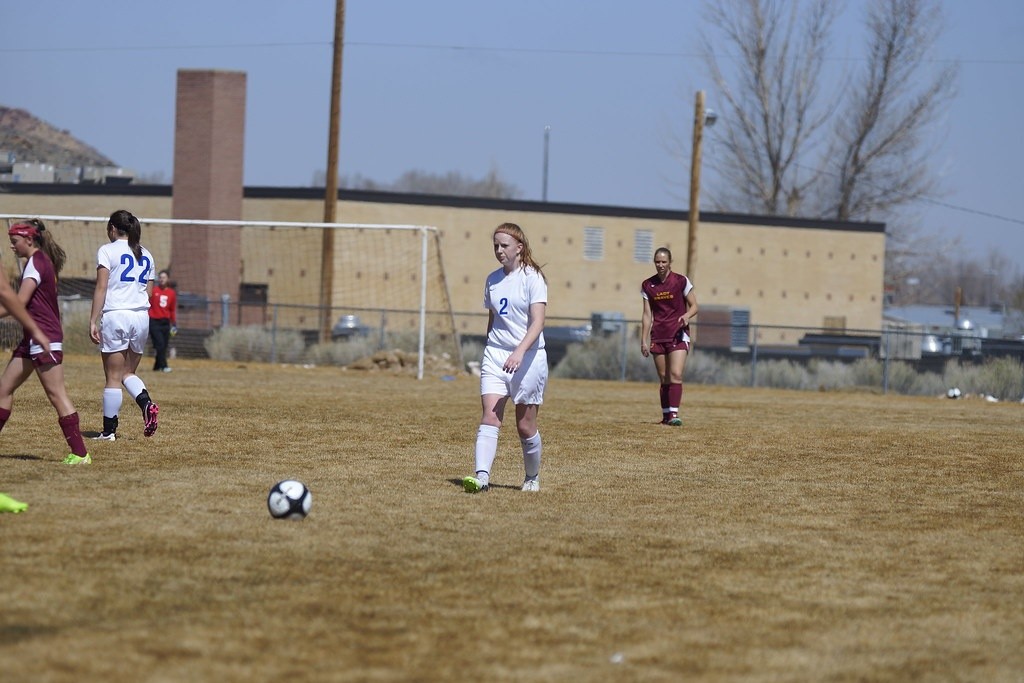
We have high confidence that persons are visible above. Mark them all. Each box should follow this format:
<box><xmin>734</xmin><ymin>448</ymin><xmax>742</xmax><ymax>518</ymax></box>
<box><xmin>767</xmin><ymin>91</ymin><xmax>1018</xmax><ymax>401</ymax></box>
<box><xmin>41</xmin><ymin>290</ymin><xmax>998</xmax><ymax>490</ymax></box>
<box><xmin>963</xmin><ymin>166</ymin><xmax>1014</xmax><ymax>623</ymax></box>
<box><xmin>148</xmin><ymin>270</ymin><xmax>177</xmax><ymax>373</ymax></box>
<box><xmin>463</xmin><ymin>223</ymin><xmax>548</xmax><ymax>494</ymax></box>
<box><xmin>0</xmin><ymin>218</ymin><xmax>91</xmax><ymax>464</ymax></box>
<box><xmin>90</xmin><ymin>210</ymin><xmax>159</xmax><ymax>442</ymax></box>
<box><xmin>0</xmin><ymin>266</ymin><xmax>50</xmax><ymax>356</ymax></box>
<box><xmin>640</xmin><ymin>247</ymin><xmax>698</xmax><ymax>426</ymax></box>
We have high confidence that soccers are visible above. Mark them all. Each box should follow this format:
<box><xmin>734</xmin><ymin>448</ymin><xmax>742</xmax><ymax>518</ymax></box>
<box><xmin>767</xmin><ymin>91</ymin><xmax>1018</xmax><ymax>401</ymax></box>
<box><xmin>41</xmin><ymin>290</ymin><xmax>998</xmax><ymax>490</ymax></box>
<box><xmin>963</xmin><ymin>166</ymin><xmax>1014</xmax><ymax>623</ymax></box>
<box><xmin>267</xmin><ymin>479</ymin><xmax>313</xmax><ymax>522</ymax></box>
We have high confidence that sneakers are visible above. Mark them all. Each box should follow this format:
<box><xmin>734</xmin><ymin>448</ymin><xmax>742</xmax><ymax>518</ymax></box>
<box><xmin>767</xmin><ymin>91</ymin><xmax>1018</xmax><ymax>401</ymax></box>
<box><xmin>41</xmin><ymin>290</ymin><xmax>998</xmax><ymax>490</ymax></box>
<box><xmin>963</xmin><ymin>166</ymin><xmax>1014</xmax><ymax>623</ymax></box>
<box><xmin>91</xmin><ymin>432</ymin><xmax>116</xmax><ymax>441</ymax></box>
<box><xmin>660</xmin><ymin>418</ymin><xmax>682</xmax><ymax>426</ymax></box>
<box><xmin>142</xmin><ymin>401</ymin><xmax>159</xmax><ymax>437</ymax></box>
<box><xmin>522</xmin><ymin>480</ymin><xmax>540</xmax><ymax>492</ymax></box>
<box><xmin>61</xmin><ymin>452</ymin><xmax>92</xmax><ymax>465</ymax></box>
<box><xmin>158</xmin><ymin>366</ymin><xmax>173</xmax><ymax>373</ymax></box>
<box><xmin>0</xmin><ymin>492</ymin><xmax>29</xmax><ymax>514</ymax></box>
<box><xmin>463</xmin><ymin>476</ymin><xmax>489</xmax><ymax>494</ymax></box>
